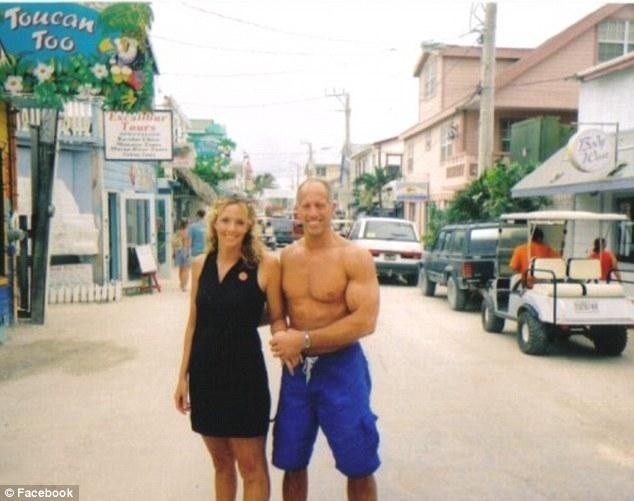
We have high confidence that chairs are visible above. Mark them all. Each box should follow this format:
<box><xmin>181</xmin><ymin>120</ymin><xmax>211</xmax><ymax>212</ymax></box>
<box><xmin>530</xmin><ymin>257</ymin><xmax>624</xmax><ymax>298</ymax></box>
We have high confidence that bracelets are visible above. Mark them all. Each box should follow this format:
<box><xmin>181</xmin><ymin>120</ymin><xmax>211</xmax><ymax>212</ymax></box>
<box><xmin>304</xmin><ymin>330</ymin><xmax>311</xmax><ymax>354</ymax></box>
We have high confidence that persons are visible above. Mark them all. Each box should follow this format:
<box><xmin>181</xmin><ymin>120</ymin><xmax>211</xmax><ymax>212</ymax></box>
<box><xmin>255</xmin><ymin>220</ymin><xmax>274</xmax><ymax>236</ymax></box>
<box><xmin>589</xmin><ymin>238</ymin><xmax>620</xmax><ymax>280</ymax></box>
<box><xmin>175</xmin><ymin>195</ymin><xmax>305</xmax><ymax>501</ymax></box>
<box><xmin>174</xmin><ymin>210</ymin><xmax>210</xmax><ymax>291</ymax></box>
<box><xmin>257</xmin><ymin>177</ymin><xmax>381</xmax><ymax>501</ymax></box>
<box><xmin>509</xmin><ymin>227</ymin><xmax>561</xmax><ymax>289</ymax></box>
